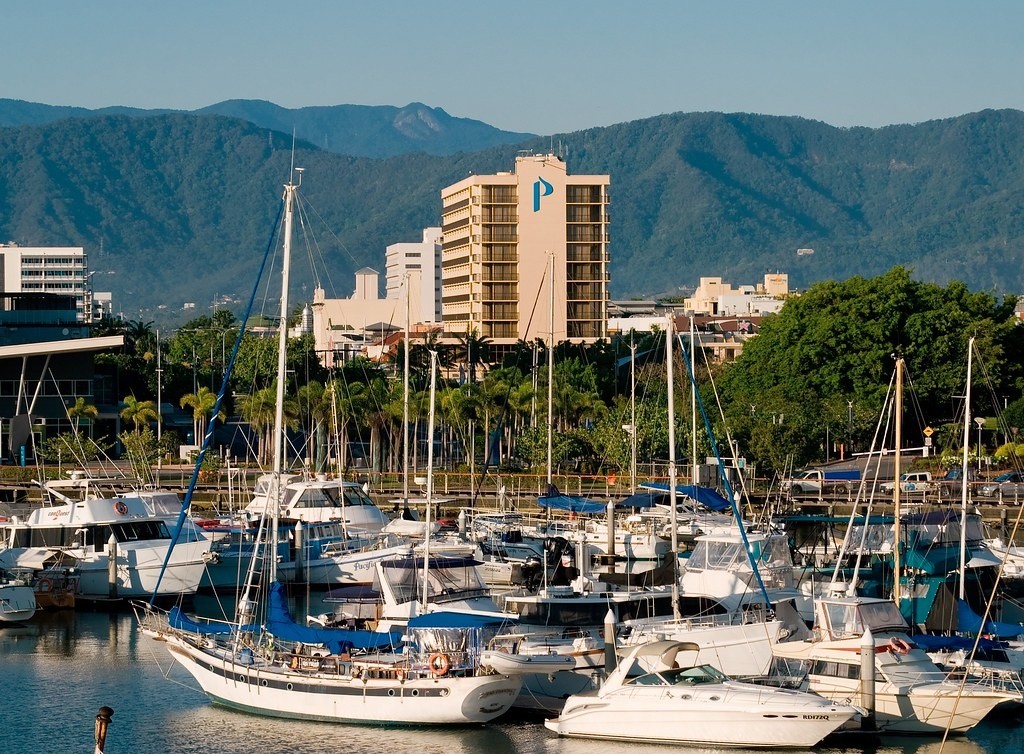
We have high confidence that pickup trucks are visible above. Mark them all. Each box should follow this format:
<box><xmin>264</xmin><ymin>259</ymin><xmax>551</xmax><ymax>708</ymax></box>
<box><xmin>925</xmin><ymin>467</ymin><xmax>987</xmax><ymax>496</ymax></box>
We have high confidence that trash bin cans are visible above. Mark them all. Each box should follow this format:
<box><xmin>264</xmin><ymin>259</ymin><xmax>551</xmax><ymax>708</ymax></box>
<box><xmin>188</xmin><ymin>449</ymin><xmax>200</xmax><ymax>465</ymax></box>
<box><xmin>607</xmin><ymin>469</ymin><xmax>616</xmax><ymax>487</ymax></box>
<box><xmin>240</xmin><ymin>648</ymin><xmax>254</xmax><ymax>664</ymax></box>
<box><xmin>689</xmin><ymin>464</ymin><xmax>710</xmax><ymax>488</ymax></box>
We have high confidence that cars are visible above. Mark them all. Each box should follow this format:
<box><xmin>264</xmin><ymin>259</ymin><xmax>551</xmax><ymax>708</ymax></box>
<box><xmin>982</xmin><ymin>470</ymin><xmax>1024</xmax><ymax>499</ymax></box>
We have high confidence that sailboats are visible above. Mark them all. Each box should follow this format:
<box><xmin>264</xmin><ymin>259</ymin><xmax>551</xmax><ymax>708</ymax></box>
<box><xmin>0</xmin><ymin>165</ymin><xmax>1024</xmax><ymax>738</ymax></box>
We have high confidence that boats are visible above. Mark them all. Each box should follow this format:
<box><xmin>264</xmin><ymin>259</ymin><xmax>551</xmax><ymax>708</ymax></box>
<box><xmin>543</xmin><ymin>639</ymin><xmax>871</xmax><ymax>750</ymax></box>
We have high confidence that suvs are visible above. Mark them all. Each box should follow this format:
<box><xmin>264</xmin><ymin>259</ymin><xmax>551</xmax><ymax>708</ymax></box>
<box><xmin>878</xmin><ymin>471</ymin><xmax>934</xmax><ymax>496</ymax></box>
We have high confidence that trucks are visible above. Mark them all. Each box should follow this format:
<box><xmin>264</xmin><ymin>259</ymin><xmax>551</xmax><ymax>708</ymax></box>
<box><xmin>778</xmin><ymin>470</ymin><xmax>862</xmax><ymax>496</ymax></box>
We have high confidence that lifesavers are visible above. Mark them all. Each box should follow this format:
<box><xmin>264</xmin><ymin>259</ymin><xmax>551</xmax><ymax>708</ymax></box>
<box><xmin>114</xmin><ymin>501</ymin><xmax>128</xmax><ymax>515</ymax></box>
<box><xmin>39</xmin><ymin>578</ymin><xmax>52</xmax><ymax>592</ymax></box>
<box><xmin>889</xmin><ymin>636</ymin><xmax>911</xmax><ymax>654</ymax></box>
<box><xmin>429</xmin><ymin>653</ymin><xmax>449</xmax><ymax>675</ymax></box>
